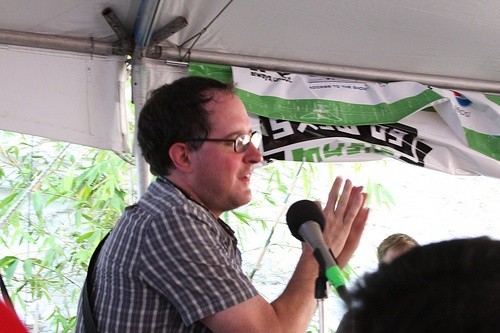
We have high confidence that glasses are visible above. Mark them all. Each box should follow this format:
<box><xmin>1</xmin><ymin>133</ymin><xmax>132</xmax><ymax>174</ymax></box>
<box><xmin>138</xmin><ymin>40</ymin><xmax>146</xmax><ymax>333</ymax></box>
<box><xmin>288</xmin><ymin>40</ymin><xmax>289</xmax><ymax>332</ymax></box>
<box><xmin>178</xmin><ymin>131</ymin><xmax>263</xmax><ymax>153</ymax></box>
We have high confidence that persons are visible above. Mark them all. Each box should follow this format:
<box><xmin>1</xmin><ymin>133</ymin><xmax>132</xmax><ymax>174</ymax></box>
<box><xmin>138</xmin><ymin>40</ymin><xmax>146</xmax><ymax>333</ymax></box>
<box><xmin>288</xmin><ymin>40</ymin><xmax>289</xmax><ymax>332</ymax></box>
<box><xmin>75</xmin><ymin>76</ymin><xmax>370</xmax><ymax>333</ymax></box>
<box><xmin>337</xmin><ymin>234</ymin><xmax>500</xmax><ymax>333</ymax></box>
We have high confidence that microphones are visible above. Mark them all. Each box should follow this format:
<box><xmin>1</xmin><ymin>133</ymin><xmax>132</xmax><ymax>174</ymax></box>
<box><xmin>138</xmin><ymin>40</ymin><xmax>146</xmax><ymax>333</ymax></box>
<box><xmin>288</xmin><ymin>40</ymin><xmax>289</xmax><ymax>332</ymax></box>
<box><xmin>286</xmin><ymin>200</ymin><xmax>354</xmax><ymax>311</ymax></box>
